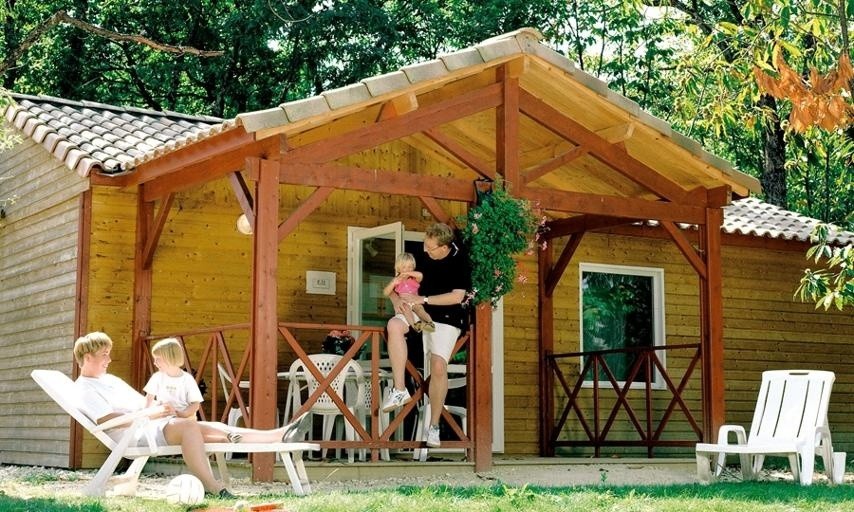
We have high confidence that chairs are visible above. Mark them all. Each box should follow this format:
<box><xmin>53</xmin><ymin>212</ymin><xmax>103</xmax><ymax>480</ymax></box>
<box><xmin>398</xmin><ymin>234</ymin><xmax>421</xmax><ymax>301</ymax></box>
<box><xmin>216</xmin><ymin>353</ymin><xmax>469</xmax><ymax>466</ymax></box>
<box><xmin>695</xmin><ymin>370</ymin><xmax>838</xmax><ymax>487</ymax></box>
<box><xmin>30</xmin><ymin>369</ymin><xmax>323</xmax><ymax>499</ymax></box>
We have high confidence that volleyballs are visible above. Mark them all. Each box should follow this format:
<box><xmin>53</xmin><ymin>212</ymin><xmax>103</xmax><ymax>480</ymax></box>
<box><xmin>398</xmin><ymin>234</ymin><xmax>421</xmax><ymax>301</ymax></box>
<box><xmin>167</xmin><ymin>475</ymin><xmax>204</xmax><ymax>507</ymax></box>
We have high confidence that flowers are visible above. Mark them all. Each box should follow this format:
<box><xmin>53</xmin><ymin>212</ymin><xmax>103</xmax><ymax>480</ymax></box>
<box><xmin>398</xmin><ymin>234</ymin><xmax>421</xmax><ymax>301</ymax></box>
<box><xmin>449</xmin><ymin>175</ymin><xmax>553</xmax><ymax>313</ymax></box>
<box><xmin>320</xmin><ymin>330</ymin><xmax>355</xmax><ymax>355</ymax></box>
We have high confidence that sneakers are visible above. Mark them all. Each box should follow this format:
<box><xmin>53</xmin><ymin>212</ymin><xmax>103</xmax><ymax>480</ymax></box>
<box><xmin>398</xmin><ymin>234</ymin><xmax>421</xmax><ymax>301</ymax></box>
<box><xmin>382</xmin><ymin>386</ymin><xmax>413</xmax><ymax>413</ymax></box>
<box><xmin>413</xmin><ymin>320</ymin><xmax>423</xmax><ymax>334</ymax></box>
<box><xmin>422</xmin><ymin>320</ymin><xmax>435</xmax><ymax>333</ymax></box>
<box><xmin>204</xmin><ymin>488</ymin><xmax>240</xmax><ymax>501</ymax></box>
<box><xmin>425</xmin><ymin>424</ymin><xmax>441</xmax><ymax>448</ymax></box>
<box><xmin>281</xmin><ymin>411</ymin><xmax>312</xmax><ymax>443</ymax></box>
<box><xmin>226</xmin><ymin>433</ymin><xmax>243</xmax><ymax>443</ymax></box>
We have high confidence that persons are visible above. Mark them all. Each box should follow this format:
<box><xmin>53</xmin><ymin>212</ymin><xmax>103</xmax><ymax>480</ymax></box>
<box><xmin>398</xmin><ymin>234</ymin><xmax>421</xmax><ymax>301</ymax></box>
<box><xmin>138</xmin><ymin>336</ymin><xmax>243</xmax><ymax>442</ymax></box>
<box><xmin>381</xmin><ymin>222</ymin><xmax>468</xmax><ymax>449</ymax></box>
<box><xmin>381</xmin><ymin>251</ymin><xmax>436</xmax><ymax>334</ymax></box>
<box><xmin>71</xmin><ymin>329</ymin><xmax>312</xmax><ymax>502</ymax></box>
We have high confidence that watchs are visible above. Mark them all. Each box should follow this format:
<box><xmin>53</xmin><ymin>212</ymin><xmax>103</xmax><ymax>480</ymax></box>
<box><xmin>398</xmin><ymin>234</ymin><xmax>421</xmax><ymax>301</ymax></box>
<box><xmin>422</xmin><ymin>293</ymin><xmax>430</xmax><ymax>305</ymax></box>
